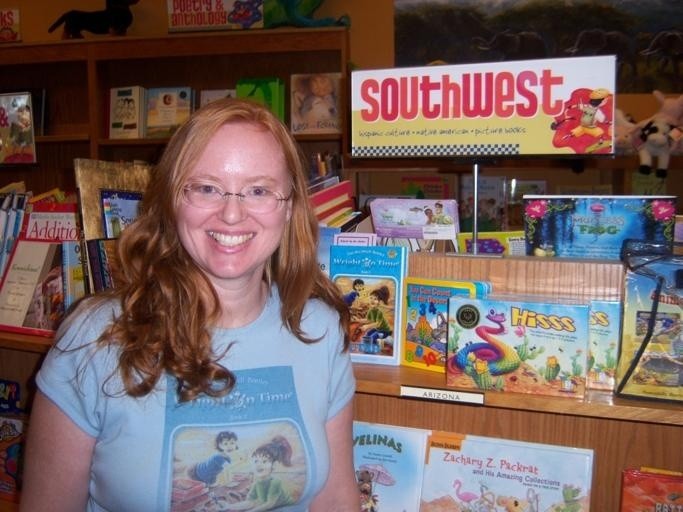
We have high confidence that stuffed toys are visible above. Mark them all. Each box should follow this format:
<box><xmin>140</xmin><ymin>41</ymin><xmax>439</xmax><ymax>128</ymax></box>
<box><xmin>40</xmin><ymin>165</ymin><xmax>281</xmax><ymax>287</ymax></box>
<box><xmin>48</xmin><ymin>0</ymin><xmax>138</xmax><ymax>42</ymax></box>
<box><xmin>634</xmin><ymin>88</ymin><xmax>682</xmax><ymax>177</ymax></box>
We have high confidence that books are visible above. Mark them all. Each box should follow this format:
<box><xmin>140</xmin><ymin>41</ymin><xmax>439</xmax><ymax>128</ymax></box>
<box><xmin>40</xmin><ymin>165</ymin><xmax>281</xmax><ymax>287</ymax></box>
<box><xmin>0</xmin><ymin>5</ymin><xmax>47</xmax><ymax>167</ymax></box>
<box><xmin>106</xmin><ymin>70</ymin><xmax>340</xmax><ymax>142</ymax></box>
<box><xmin>166</xmin><ymin>0</ymin><xmax>264</xmax><ymax>33</ymax></box>
<box><xmin>0</xmin><ymin>177</ymin><xmax>683</xmax><ymax>510</ymax></box>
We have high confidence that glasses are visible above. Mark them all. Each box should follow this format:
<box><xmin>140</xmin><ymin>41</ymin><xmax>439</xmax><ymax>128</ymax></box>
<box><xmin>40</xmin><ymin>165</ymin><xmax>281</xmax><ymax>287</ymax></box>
<box><xmin>178</xmin><ymin>181</ymin><xmax>294</xmax><ymax>215</ymax></box>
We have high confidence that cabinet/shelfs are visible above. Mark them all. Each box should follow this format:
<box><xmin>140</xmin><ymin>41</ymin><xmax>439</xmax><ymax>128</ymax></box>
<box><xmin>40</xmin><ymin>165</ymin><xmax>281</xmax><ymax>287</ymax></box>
<box><xmin>1</xmin><ymin>1</ymin><xmax>394</xmax><ymax>219</ymax></box>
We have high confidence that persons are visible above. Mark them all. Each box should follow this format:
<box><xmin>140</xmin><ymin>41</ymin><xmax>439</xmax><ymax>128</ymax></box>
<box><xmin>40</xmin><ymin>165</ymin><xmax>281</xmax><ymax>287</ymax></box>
<box><xmin>18</xmin><ymin>98</ymin><xmax>360</xmax><ymax>511</ymax></box>
